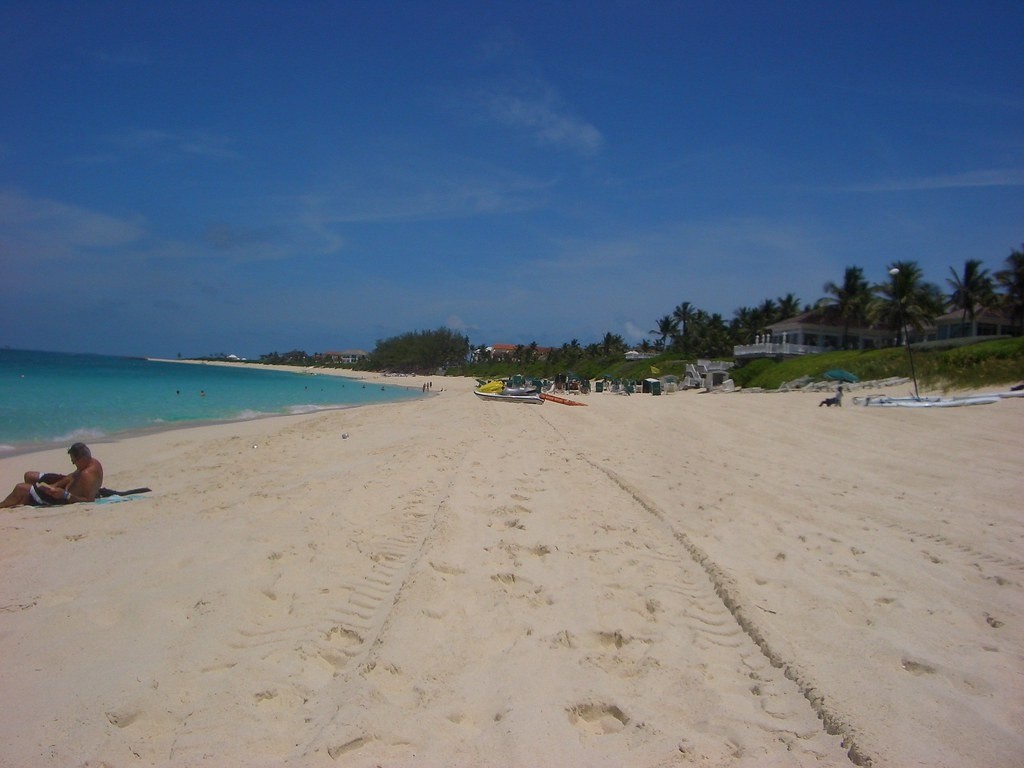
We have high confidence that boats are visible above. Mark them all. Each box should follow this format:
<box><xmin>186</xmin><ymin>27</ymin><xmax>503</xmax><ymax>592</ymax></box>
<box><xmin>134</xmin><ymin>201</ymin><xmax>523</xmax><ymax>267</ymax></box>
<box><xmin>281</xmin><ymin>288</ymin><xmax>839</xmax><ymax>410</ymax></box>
<box><xmin>474</xmin><ymin>391</ymin><xmax>545</xmax><ymax>405</ymax></box>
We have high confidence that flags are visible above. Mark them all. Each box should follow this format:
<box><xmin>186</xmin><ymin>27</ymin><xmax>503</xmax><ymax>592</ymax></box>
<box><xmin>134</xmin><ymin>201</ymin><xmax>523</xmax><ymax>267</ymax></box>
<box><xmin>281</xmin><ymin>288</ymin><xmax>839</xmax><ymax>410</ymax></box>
<box><xmin>651</xmin><ymin>367</ymin><xmax>659</xmax><ymax>374</ymax></box>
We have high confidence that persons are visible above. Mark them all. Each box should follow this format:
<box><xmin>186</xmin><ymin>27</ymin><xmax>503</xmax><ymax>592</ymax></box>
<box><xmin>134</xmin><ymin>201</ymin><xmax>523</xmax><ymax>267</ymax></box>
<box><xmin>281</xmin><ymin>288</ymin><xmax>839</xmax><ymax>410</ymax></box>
<box><xmin>819</xmin><ymin>386</ymin><xmax>843</xmax><ymax>407</ymax></box>
<box><xmin>176</xmin><ymin>390</ymin><xmax>180</xmax><ymax>394</ymax></box>
<box><xmin>304</xmin><ymin>381</ymin><xmax>432</xmax><ymax>393</ymax></box>
<box><xmin>200</xmin><ymin>390</ymin><xmax>205</xmax><ymax>396</ymax></box>
<box><xmin>0</xmin><ymin>442</ymin><xmax>103</xmax><ymax>508</ymax></box>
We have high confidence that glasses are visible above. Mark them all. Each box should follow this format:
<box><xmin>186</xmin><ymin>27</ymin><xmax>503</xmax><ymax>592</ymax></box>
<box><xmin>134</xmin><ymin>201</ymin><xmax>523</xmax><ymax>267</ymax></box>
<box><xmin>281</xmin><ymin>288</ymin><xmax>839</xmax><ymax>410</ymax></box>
<box><xmin>73</xmin><ymin>456</ymin><xmax>80</xmax><ymax>464</ymax></box>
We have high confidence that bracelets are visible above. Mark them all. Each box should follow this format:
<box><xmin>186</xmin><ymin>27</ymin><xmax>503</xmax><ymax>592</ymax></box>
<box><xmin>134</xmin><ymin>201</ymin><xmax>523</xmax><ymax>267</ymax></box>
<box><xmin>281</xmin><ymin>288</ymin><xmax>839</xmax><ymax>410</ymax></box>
<box><xmin>64</xmin><ymin>492</ymin><xmax>72</xmax><ymax>499</ymax></box>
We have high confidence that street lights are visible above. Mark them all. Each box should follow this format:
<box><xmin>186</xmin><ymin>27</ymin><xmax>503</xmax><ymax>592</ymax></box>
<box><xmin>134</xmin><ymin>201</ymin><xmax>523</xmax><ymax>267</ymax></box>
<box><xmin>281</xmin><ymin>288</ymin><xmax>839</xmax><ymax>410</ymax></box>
<box><xmin>889</xmin><ymin>266</ymin><xmax>920</xmax><ymax>403</ymax></box>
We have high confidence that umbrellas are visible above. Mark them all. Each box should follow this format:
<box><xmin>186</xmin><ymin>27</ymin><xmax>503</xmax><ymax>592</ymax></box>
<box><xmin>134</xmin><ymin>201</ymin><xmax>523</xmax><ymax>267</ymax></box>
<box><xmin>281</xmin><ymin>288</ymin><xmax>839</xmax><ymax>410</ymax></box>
<box><xmin>823</xmin><ymin>368</ymin><xmax>858</xmax><ymax>389</ymax></box>
